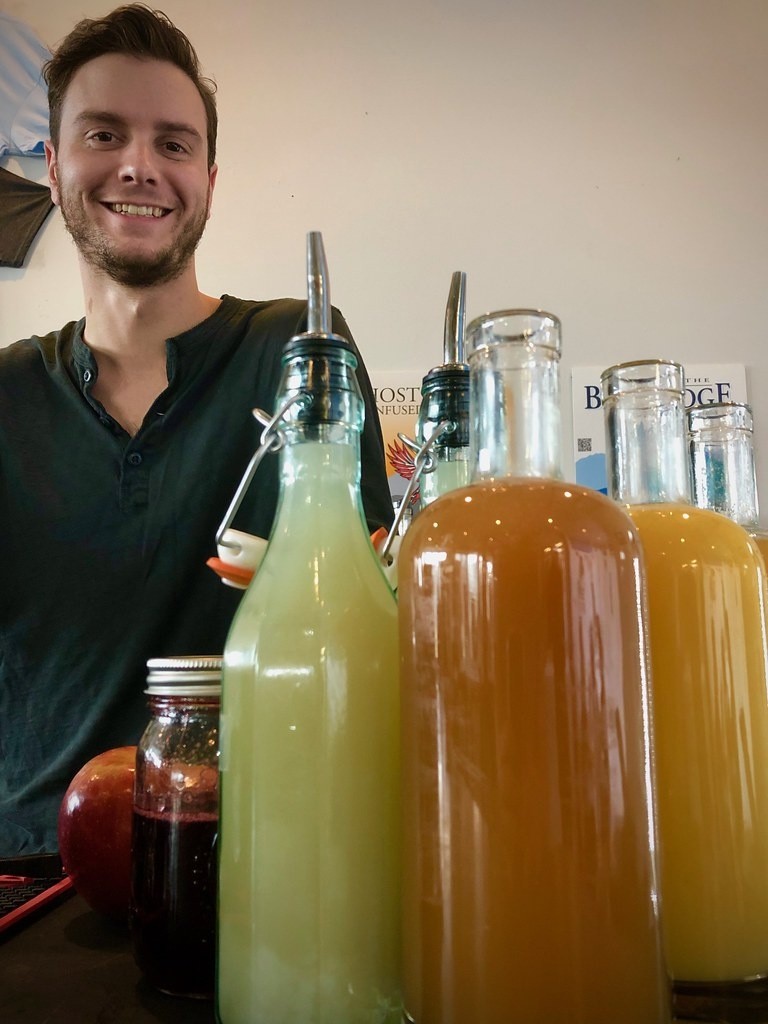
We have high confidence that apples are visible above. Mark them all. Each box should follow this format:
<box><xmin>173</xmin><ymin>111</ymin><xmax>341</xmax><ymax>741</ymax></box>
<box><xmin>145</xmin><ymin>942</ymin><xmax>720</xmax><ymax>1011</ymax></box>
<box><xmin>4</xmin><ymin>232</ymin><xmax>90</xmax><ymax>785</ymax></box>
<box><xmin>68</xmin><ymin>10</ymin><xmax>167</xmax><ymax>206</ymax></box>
<box><xmin>53</xmin><ymin>747</ymin><xmax>219</xmax><ymax>926</ymax></box>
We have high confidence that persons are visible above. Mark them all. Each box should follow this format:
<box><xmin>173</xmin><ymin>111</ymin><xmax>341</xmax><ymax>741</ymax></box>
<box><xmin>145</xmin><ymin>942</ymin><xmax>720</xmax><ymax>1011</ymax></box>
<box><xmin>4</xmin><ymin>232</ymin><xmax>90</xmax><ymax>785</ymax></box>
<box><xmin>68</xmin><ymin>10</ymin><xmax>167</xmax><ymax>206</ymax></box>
<box><xmin>0</xmin><ymin>4</ymin><xmax>398</xmax><ymax>859</ymax></box>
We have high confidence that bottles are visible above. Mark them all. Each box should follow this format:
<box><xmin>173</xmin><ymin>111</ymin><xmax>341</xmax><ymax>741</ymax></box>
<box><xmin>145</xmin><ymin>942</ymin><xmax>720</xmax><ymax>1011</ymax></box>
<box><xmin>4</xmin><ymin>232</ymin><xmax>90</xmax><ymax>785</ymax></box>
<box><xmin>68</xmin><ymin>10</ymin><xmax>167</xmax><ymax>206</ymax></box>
<box><xmin>133</xmin><ymin>654</ymin><xmax>225</xmax><ymax>1003</ymax></box>
<box><xmin>599</xmin><ymin>359</ymin><xmax>768</xmax><ymax>998</ymax></box>
<box><xmin>394</xmin><ymin>311</ymin><xmax>673</xmax><ymax>1024</ymax></box>
<box><xmin>681</xmin><ymin>402</ymin><xmax>768</xmax><ymax>572</ymax></box>
<box><xmin>205</xmin><ymin>230</ymin><xmax>403</xmax><ymax>1024</ymax></box>
<box><xmin>367</xmin><ymin>269</ymin><xmax>501</xmax><ymax>604</ymax></box>
